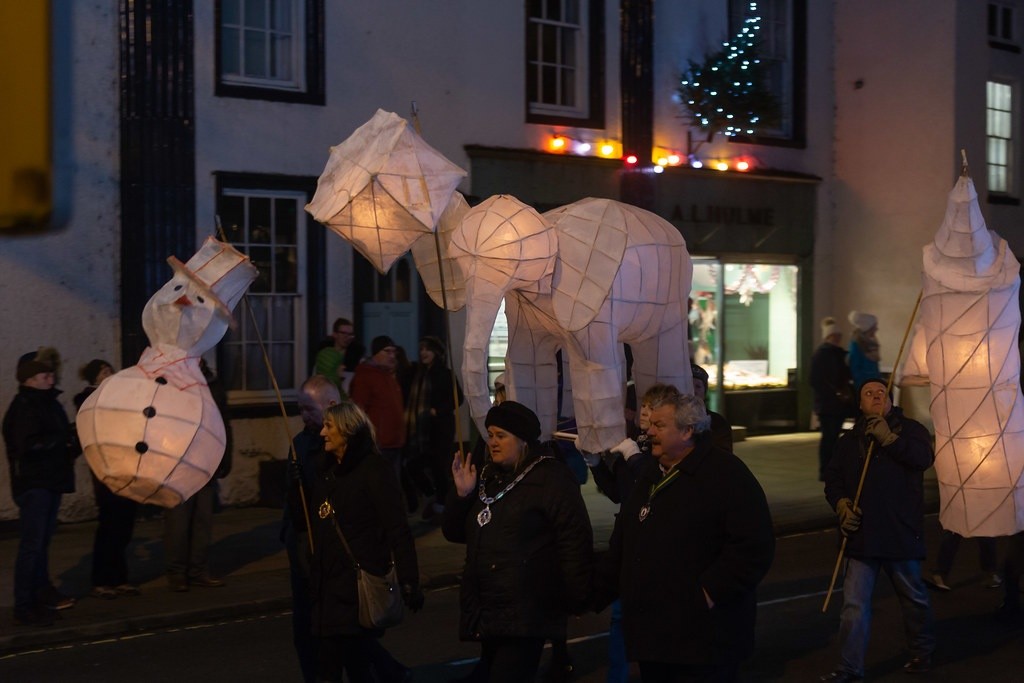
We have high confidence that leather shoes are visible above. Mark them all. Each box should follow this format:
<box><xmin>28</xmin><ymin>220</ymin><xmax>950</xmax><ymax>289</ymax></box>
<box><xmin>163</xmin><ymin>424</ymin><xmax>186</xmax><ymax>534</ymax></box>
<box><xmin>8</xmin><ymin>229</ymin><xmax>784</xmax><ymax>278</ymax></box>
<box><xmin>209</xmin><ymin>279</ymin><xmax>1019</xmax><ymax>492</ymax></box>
<box><xmin>190</xmin><ymin>574</ymin><xmax>228</xmax><ymax>587</ymax></box>
<box><xmin>168</xmin><ymin>578</ymin><xmax>190</xmax><ymax>591</ymax></box>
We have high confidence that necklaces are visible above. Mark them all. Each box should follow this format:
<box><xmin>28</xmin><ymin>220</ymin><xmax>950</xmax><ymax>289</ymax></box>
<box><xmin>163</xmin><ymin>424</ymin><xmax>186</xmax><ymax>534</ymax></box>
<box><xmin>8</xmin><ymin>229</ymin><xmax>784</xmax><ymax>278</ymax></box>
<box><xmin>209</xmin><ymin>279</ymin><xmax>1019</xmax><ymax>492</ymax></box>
<box><xmin>319</xmin><ymin>494</ymin><xmax>330</xmax><ymax>518</ymax></box>
<box><xmin>640</xmin><ymin>470</ymin><xmax>682</xmax><ymax>522</ymax></box>
<box><xmin>477</xmin><ymin>455</ymin><xmax>556</xmax><ymax>528</ymax></box>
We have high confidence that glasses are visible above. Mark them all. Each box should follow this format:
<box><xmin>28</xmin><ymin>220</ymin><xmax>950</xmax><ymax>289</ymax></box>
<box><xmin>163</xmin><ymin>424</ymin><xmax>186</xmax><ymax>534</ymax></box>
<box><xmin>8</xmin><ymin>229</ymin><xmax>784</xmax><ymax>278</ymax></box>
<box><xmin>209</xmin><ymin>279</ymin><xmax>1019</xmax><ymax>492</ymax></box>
<box><xmin>383</xmin><ymin>349</ymin><xmax>399</xmax><ymax>355</ymax></box>
<box><xmin>334</xmin><ymin>330</ymin><xmax>356</xmax><ymax>340</ymax></box>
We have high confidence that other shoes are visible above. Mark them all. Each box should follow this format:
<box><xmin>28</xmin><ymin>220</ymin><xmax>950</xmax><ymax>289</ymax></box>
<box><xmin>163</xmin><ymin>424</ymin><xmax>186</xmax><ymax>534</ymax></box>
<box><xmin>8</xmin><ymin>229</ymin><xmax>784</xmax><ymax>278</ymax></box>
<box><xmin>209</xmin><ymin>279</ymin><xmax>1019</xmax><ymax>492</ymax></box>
<box><xmin>13</xmin><ymin>609</ymin><xmax>53</xmax><ymax>627</ymax></box>
<box><xmin>40</xmin><ymin>589</ymin><xmax>74</xmax><ymax>609</ymax></box>
<box><xmin>113</xmin><ymin>584</ymin><xmax>138</xmax><ymax>597</ymax></box>
<box><xmin>902</xmin><ymin>655</ymin><xmax>932</xmax><ymax>672</ymax></box>
<box><xmin>928</xmin><ymin>575</ymin><xmax>951</xmax><ymax>592</ymax></box>
<box><xmin>91</xmin><ymin>587</ymin><xmax>120</xmax><ymax>599</ymax></box>
<box><xmin>818</xmin><ymin>670</ymin><xmax>865</xmax><ymax>683</ymax></box>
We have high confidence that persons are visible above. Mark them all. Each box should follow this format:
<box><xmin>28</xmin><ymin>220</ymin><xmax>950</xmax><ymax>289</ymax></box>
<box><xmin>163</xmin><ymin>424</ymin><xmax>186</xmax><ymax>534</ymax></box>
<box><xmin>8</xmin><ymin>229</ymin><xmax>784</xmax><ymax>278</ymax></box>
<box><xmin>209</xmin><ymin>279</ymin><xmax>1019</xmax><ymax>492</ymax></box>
<box><xmin>314</xmin><ymin>317</ymin><xmax>465</xmax><ymax>516</ymax></box>
<box><xmin>807</xmin><ymin>309</ymin><xmax>882</xmax><ymax>486</ymax></box>
<box><xmin>163</xmin><ymin>356</ymin><xmax>233</xmax><ymax>592</ymax></box>
<box><xmin>72</xmin><ymin>360</ymin><xmax>140</xmax><ymax>598</ymax></box>
<box><xmin>2</xmin><ymin>348</ymin><xmax>83</xmax><ymax>627</ymax></box>
<box><xmin>278</xmin><ymin>375</ymin><xmax>423</xmax><ymax>683</ymax></box>
<box><xmin>441</xmin><ymin>382</ymin><xmax>776</xmax><ymax>683</ymax></box>
<box><xmin>927</xmin><ymin>530</ymin><xmax>1024</xmax><ymax>616</ymax></box>
<box><xmin>820</xmin><ymin>375</ymin><xmax>935</xmax><ymax>683</ymax></box>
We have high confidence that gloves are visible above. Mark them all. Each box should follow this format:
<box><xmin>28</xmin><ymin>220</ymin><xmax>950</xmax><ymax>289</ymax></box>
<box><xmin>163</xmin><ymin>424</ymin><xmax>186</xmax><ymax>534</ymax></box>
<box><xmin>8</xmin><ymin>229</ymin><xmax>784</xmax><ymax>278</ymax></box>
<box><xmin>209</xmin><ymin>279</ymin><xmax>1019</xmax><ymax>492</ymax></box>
<box><xmin>283</xmin><ymin>461</ymin><xmax>304</xmax><ymax>484</ymax></box>
<box><xmin>835</xmin><ymin>497</ymin><xmax>863</xmax><ymax>538</ymax></box>
<box><xmin>405</xmin><ymin>586</ymin><xmax>425</xmax><ymax>614</ymax></box>
<box><xmin>864</xmin><ymin>414</ymin><xmax>899</xmax><ymax>445</ymax></box>
<box><xmin>610</xmin><ymin>438</ymin><xmax>642</xmax><ymax>462</ymax></box>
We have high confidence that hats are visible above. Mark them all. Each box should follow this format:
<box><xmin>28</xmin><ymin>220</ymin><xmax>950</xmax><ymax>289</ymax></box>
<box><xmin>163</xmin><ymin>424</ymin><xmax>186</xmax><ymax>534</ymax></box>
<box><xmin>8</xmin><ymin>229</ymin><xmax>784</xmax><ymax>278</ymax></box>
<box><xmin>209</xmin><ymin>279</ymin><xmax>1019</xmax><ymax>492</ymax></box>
<box><xmin>371</xmin><ymin>336</ymin><xmax>397</xmax><ymax>356</ymax></box>
<box><xmin>421</xmin><ymin>335</ymin><xmax>444</xmax><ymax>356</ymax></box>
<box><xmin>484</xmin><ymin>401</ymin><xmax>541</xmax><ymax>448</ymax></box>
<box><xmin>17</xmin><ymin>347</ymin><xmax>57</xmax><ymax>384</ymax></box>
<box><xmin>79</xmin><ymin>359</ymin><xmax>110</xmax><ymax>385</ymax></box>
<box><xmin>821</xmin><ymin>317</ymin><xmax>844</xmax><ymax>340</ymax></box>
<box><xmin>848</xmin><ymin>311</ymin><xmax>876</xmax><ymax>334</ymax></box>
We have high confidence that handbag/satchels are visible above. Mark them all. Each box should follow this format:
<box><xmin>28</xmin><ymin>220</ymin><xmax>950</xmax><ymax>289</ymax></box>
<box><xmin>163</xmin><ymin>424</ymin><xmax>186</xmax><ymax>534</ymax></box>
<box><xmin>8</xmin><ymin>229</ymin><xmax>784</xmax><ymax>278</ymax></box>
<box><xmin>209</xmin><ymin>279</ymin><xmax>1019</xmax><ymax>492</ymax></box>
<box><xmin>355</xmin><ymin>566</ymin><xmax>405</xmax><ymax>628</ymax></box>
